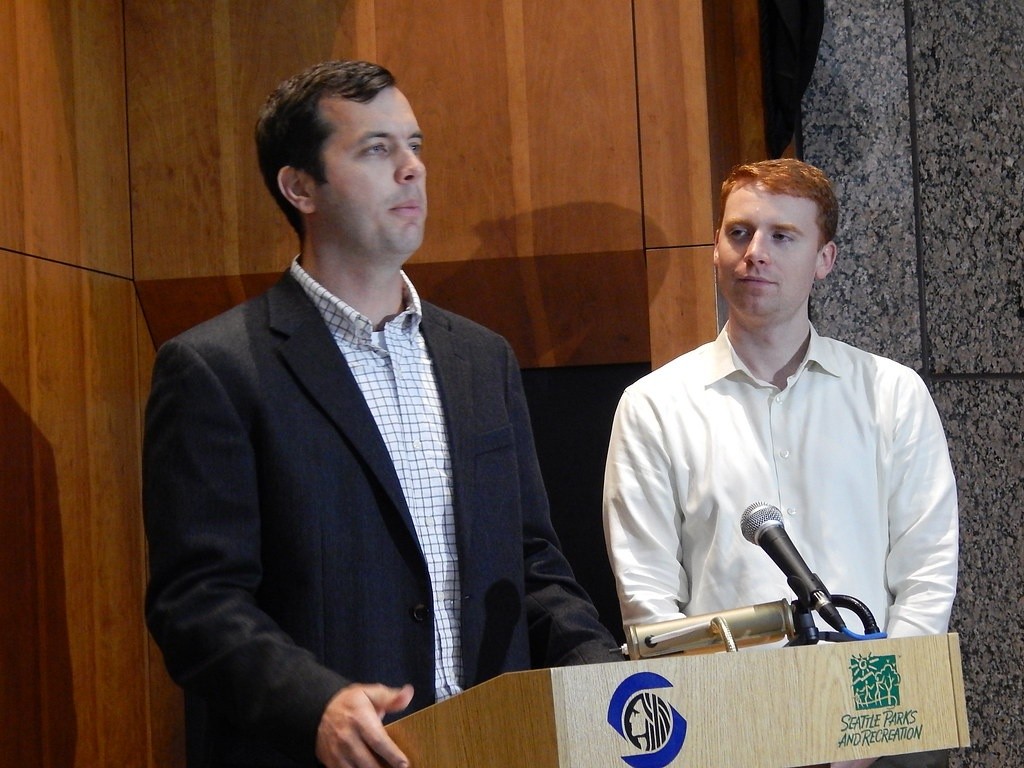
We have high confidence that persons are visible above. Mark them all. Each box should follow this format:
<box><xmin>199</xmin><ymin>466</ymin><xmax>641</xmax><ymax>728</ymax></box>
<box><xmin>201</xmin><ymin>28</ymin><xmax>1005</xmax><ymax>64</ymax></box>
<box><xmin>604</xmin><ymin>156</ymin><xmax>960</xmax><ymax>768</ymax></box>
<box><xmin>141</xmin><ymin>60</ymin><xmax>623</xmax><ymax>768</ymax></box>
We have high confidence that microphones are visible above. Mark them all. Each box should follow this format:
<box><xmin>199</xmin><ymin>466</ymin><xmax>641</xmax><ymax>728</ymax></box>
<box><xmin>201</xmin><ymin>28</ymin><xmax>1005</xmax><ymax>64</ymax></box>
<box><xmin>740</xmin><ymin>502</ymin><xmax>845</xmax><ymax>633</ymax></box>
